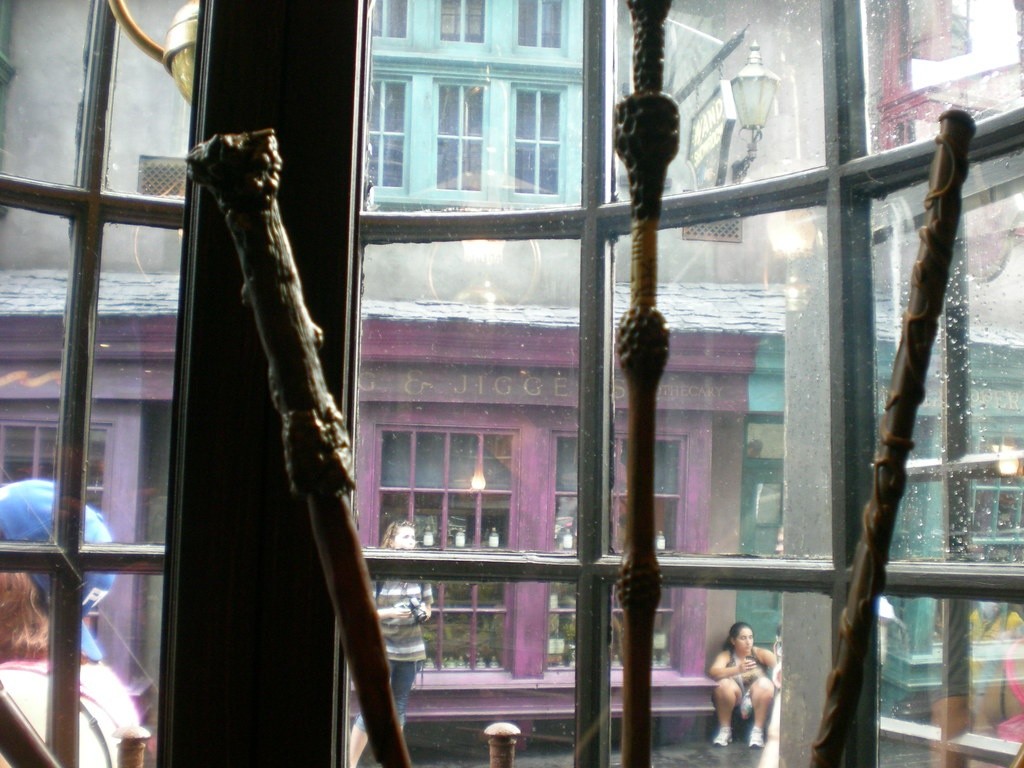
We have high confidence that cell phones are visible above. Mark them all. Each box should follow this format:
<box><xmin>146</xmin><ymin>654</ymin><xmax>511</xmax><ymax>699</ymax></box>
<box><xmin>746</xmin><ymin>656</ymin><xmax>754</xmax><ymax>662</ymax></box>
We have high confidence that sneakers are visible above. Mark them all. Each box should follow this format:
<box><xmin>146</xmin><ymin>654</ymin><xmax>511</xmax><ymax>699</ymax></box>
<box><xmin>713</xmin><ymin>727</ymin><xmax>732</xmax><ymax>745</ymax></box>
<box><xmin>749</xmin><ymin>727</ymin><xmax>765</xmax><ymax>747</ymax></box>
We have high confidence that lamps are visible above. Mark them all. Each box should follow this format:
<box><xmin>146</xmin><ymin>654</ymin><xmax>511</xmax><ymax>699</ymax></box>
<box><xmin>563</xmin><ymin>527</ymin><xmax>572</xmax><ymax>549</ymax></box>
<box><xmin>728</xmin><ymin>36</ymin><xmax>780</xmax><ymax>185</ymax></box>
<box><xmin>488</xmin><ymin>526</ymin><xmax>499</xmax><ymax>546</ymax></box>
<box><xmin>422</xmin><ymin>524</ymin><xmax>436</xmax><ymax>545</ymax></box>
<box><xmin>102</xmin><ymin>0</ymin><xmax>200</xmax><ymax>106</ymax></box>
<box><xmin>454</xmin><ymin>526</ymin><xmax>466</xmax><ymax>546</ymax></box>
<box><xmin>656</xmin><ymin>531</ymin><xmax>666</xmax><ymax>550</ymax></box>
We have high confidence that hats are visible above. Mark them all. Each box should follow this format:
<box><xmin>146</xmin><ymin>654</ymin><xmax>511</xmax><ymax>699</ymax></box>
<box><xmin>0</xmin><ymin>480</ymin><xmax>114</xmax><ymax>660</ymax></box>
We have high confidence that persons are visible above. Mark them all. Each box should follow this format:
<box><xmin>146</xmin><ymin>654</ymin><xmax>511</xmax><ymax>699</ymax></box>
<box><xmin>708</xmin><ymin>622</ymin><xmax>779</xmax><ymax>751</ymax></box>
<box><xmin>926</xmin><ymin>598</ymin><xmax>1024</xmax><ymax>767</ymax></box>
<box><xmin>347</xmin><ymin>518</ymin><xmax>436</xmax><ymax>767</ymax></box>
<box><xmin>0</xmin><ymin>478</ymin><xmax>148</xmax><ymax>768</ymax></box>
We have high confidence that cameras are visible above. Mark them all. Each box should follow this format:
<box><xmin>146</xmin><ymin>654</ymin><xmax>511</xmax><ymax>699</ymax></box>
<box><xmin>399</xmin><ymin>596</ymin><xmax>427</xmax><ymax>623</ymax></box>
